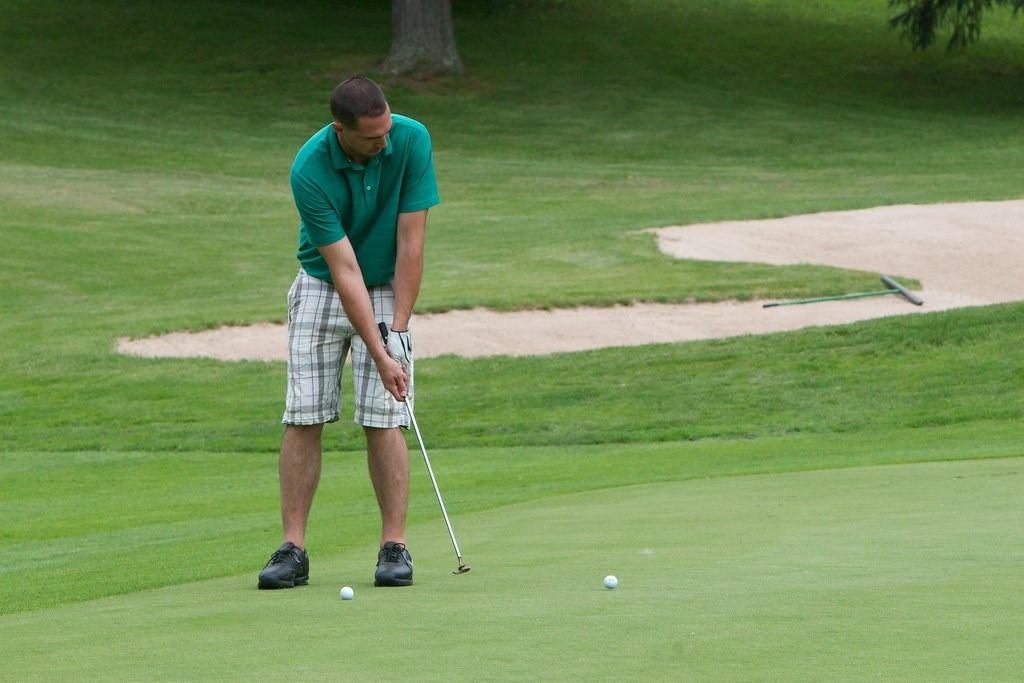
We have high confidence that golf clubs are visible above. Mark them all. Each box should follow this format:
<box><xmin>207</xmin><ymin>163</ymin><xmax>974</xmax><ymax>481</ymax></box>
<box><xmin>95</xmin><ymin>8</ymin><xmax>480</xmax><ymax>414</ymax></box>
<box><xmin>378</xmin><ymin>318</ymin><xmax>471</xmax><ymax>575</ymax></box>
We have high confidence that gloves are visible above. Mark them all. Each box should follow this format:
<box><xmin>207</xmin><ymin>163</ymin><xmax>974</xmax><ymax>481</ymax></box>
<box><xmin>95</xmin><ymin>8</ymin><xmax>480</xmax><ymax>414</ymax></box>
<box><xmin>387</xmin><ymin>327</ymin><xmax>412</xmax><ymax>375</ymax></box>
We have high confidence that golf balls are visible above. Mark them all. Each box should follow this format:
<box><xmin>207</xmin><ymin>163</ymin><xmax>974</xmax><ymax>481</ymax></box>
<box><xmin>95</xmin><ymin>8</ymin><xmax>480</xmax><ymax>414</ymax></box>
<box><xmin>340</xmin><ymin>587</ymin><xmax>354</xmax><ymax>600</ymax></box>
<box><xmin>604</xmin><ymin>574</ymin><xmax>618</xmax><ymax>589</ymax></box>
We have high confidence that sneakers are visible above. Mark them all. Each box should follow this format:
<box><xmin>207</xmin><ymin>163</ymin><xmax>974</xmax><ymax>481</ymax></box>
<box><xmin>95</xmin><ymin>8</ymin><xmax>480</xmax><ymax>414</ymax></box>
<box><xmin>374</xmin><ymin>541</ymin><xmax>413</xmax><ymax>587</ymax></box>
<box><xmin>257</xmin><ymin>542</ymin><xmax>309</xmax><ymax>590</ymax></box>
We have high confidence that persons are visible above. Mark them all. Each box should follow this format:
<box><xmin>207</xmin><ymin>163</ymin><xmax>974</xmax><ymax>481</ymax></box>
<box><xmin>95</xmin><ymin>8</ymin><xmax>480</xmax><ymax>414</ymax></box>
<box><xmin>257</xmin><ymin>78</ymin><xmax>440</xmax><ymax>585</ymax></box>
